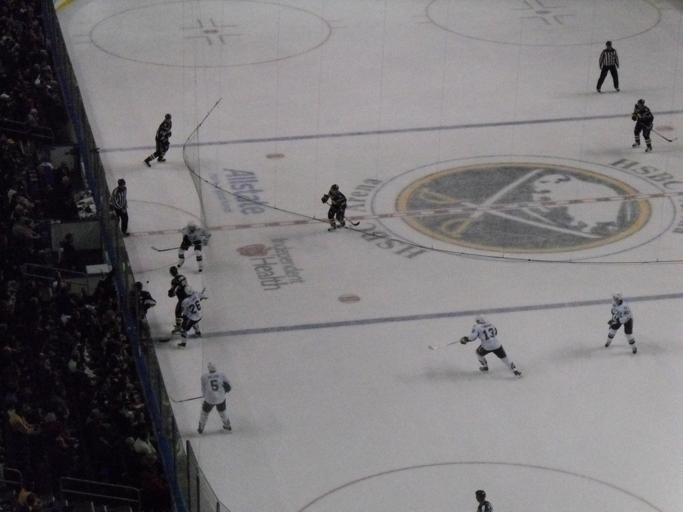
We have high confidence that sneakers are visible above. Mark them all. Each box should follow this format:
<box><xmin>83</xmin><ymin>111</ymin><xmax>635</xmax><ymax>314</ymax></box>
<box><xmin>196</xmin><ymin>428</ymin><xmax>204</xmax><ymax>433</ymax></box>
<box><xmin>122</xmin><ymin>231</ymin><xmax>130</xmax><ymax>236</ymax></box>
<box><xmin>514</xmin><ymin>371</ymin><xmax>522</xmax><ymax>376</ymax></box>
<box><xmin>194</xmin><ymin>331</ymin><xmax>201</xmax><ymax>335</ymax></box>
<box><xmin>329</xmin><ymin>221</ymin><xmax>345</xmax><ymax>230</ymax></box>
<box><xmin>199</xmin><ymin>269</ymin><xmax>202</xmax><ymax>272</ymax></box>
<box><xmin>223</xmin><ymin>425</ymin><xmax>231</xmax><ymax>431</ymax></box>
<box><xmin>632</xmin><ymin>345</ymin><xmax>638</xmax><ymax>354</ymax></box>
<box><xmin>632</xmin><ymin>142</ymin><xmax>652</xmax><ymax>152</ymax></box>
<box><xmin>178</xmin><ymin>343</ymin><xmax>186</xmax><ymax>347</ymax></box>
<box><xmin>614</xmin><ymin>87</ymin><xmax>619</xmax><ymax>91</ymax></box>
<box><xmin>605</xmin><ymin>341</ymin><xmax>612</xmax><ymax>347</ymax></box>
<box><xmin>178</xmin><ymin>261</ymin><xmax>184</xmax><ymax>267</ymax></box>
<box><xmin>479</xmin><ymin>366</ymin><xmax>488</xmax><ymax>371</ymax></box>
<box><xmin>597</xmin><ymin>88</ymin><xmax>601</xmax><ymax>92</ymax></box>
<box><xmin>157</xmin><ymin>157</ymin><xmax>166</xmax><ymax>162</ymax></box>
<box><xmin>145</xmin><ymin>159</ymin><xmax>151</xmax><ymax>167</ymax></box>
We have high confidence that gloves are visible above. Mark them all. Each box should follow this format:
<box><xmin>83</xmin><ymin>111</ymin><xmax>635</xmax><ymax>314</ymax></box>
<box><xmin>321</xmin><ymin>193</ymin><xmax>329</xmax><ymax>203</ymax></box>
<box><xmin>460</xmin><ymin>336</ymin><xmax>469</xmax><ymax>344</ymax></box>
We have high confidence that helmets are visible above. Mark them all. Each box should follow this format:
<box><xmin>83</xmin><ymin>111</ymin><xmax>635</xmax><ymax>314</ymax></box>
<box><xmin>188</xmin><ymin>220</ymin><xmax>196</xmax><ymax>229</ymax></box>
<box><xmin>613</xmin><ymin>291</ymin><xmax>623</xmax><ymax>301</ymax></box>
<box><xmin>169</xmin><ymin>266</ymin><xmax>177</xmax><ymax>275</ymax></box>
<box><xmin>118</xmin><ymin>179</ymin><xmax>126</xmax><ymax>186</ymax></box>
<box><xmin>331</xmin><ymin>184</ymin><xmax>340</xmax><ymax>191</ymax></box>
<box><xmin>475</xmin><ymin>490</ymin><xmax>487</xmax><ymax>500</ymax></box>
<box><xmin>605</xmin><ymin>40</ymin><xmax>612</xmax><ymax>46</ymax></box>
<box><xmin>475</xmin><ymin>313</ymin><xmax>486</xmax><ymax>321</ymax></box>
<box><xmin>638</xmin><ymin>99</ymin><xmax>645</xmax><ymax>105</ymax></box>
<box><xmin>165</xmin><ymin>113</ymin><xmax>172</xmax><ymax>120</ymax></box>
<box><xmin>207</xmin><ymin>361</ymin><xmax>217</xmax><ymax>371</ymax></box>
<box><xmin>185</xmin><ymin>286</ymin><xmax>193</xmax><ymax>294</ymax></box>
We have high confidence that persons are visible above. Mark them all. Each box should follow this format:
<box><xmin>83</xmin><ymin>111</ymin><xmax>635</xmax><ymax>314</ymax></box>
<box><xmin>460</xmin><ymin>314</ymin><xmax>522</xmax><ymax>377</ymax></box>
<box><xmin>605</xmin><ymin>293</ymin><xmax>637</xmax><ymax>354</ymax></box>
<box><xmin>177</xmin><ymin>220</ymin><xmax>212</xmax><ymax>273</ymax></box>
<box><xmin>596</xmin><ymin>40</ymin><xmax>620</xmax><ymax>92</ymax></box>
<box><xmin>1</xmin><ymin>278</ymin><xmax>158</xmax><ymax>512</ymax></box>
<box><xmin>321</xmin><ymin>184</ymin><xmax>347</xmax><ymax>231</ymax></box>
<box><xmin>144</xmin><ymin>114</ymin><xmax>172</xmax><ymax>167</ymax></box>
<box><xmin>631</xmin><ymin>99</ymin><xmax>654</xmax><ymax>152</ymax></box>
<box><xmin>197</xmin><ymin>360</ymin><xmax>233</xmax><ymax>434</ymax></box>
<box><xmin>0</xmin><ymin>1</ymin><xmax>83</xmax><ymax>283</ymax></box>
<box><xmin>111</xmin><ymin>179</ymin><xmax>130</xmax><ymax>236</ymax></box>
<box><xmin>168</xmin><ymin>266</ymin><xmax>209</xmax><ymax>348</ymax></box>
<box><xmin>475</xmin><ymin>490</ymin><xmax>493</xmax><ymax>512</ymax></box>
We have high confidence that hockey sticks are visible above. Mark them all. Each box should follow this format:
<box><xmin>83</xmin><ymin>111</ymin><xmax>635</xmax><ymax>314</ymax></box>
<box><xmin>200</xmin><ymin>297</ymin><xmax>208</xmax><ymax>301</ymax></box>
<box><xmin>170</xmin><ymin>394</ymin><xmax>203</xmax><ymax>403</ymax></box>
<box><xmin>151</xmin><ymin>245</ymin><xmax>204</xmax><ymax>251</ymax></box>
<box><xmin>632</xmin><ymin>113</ymin><xmax>678</xmax><ymax>142</ymax></box>
<box><xmin>326</xmin><ymin>201</ymin><xmax>360</xmax><ymax>226</ymax></box>
<box><xmin>428</xmin><ymin>341</ymin><xmax>461</xmax><ymax>351</ymax></box>
